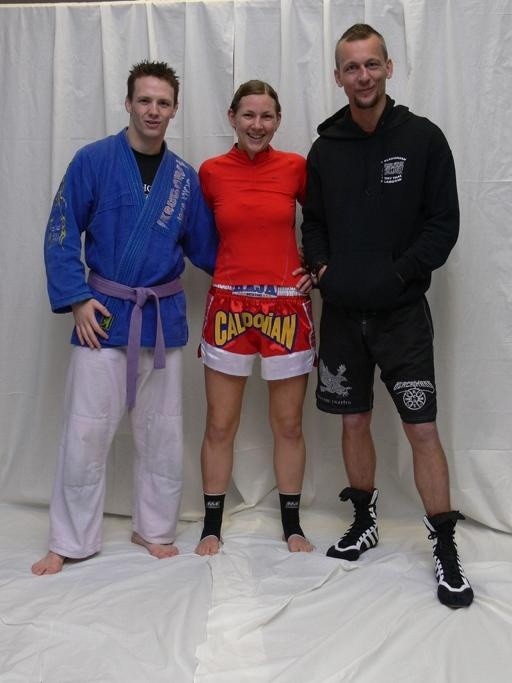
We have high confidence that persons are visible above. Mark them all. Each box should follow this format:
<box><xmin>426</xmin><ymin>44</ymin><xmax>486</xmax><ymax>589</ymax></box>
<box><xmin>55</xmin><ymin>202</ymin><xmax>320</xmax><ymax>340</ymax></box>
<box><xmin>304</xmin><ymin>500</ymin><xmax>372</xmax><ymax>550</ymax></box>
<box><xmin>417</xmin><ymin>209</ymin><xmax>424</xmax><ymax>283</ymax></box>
<box><xmin>193</xmin><ymin>80</ymin><xmax>317</xmax><ymax>557</ymax></box>
<box><xmin>32</xmin><ymin>56</ymin><xmax>305</xmax><ymax>575</ymax></box>
<box><xmin>300</xmin><ymin>24</ymin><xmax>476</xmax><ymax>609</ymax></box>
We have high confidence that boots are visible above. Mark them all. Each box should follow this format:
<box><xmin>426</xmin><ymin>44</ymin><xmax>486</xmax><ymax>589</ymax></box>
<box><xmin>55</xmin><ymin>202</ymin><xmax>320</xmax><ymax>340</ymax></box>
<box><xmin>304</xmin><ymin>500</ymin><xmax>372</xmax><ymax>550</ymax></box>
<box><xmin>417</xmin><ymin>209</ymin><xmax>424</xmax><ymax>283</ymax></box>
<box><xmin>422</xmin><ymin>510</ymin><xmax>474</xmax><ymax>608</ymax></box>
<box><xmin>328</xmin><ymin>486</ymin><xmax>379</xmax><ymax>560</ymax></box>
